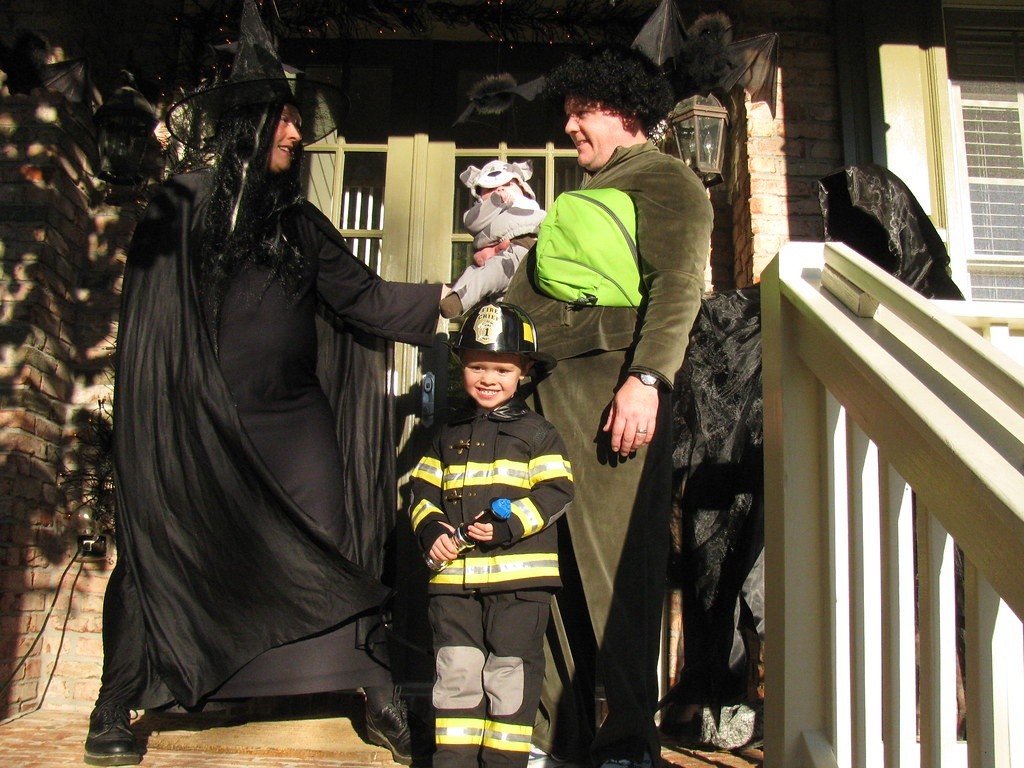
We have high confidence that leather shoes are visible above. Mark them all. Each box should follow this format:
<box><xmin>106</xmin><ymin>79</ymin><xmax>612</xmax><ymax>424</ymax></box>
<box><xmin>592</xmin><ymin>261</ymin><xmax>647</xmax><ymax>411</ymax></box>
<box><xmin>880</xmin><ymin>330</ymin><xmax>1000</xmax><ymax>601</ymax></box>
<box><xmin>84</xmin><ymin>698</ymin><xmax>143</xmax><ymax>764</ymax></box>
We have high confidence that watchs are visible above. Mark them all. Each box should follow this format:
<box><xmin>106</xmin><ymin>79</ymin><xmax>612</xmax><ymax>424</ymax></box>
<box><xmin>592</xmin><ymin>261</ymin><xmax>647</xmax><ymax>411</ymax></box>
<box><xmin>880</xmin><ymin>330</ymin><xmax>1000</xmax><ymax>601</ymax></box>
<box><xmin>630</xmin><ymin>372</ymin><xmax>660</xmax><ymax>387</ymax></box>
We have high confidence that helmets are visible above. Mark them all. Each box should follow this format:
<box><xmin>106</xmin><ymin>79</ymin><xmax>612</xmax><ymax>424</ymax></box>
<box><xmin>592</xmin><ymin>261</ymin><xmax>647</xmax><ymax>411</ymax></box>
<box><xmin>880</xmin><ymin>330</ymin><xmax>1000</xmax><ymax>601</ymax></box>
<box><xmin>443</xmin><ymin>298</ymin><xmax>559</xmax><ymax>376</ymax></box>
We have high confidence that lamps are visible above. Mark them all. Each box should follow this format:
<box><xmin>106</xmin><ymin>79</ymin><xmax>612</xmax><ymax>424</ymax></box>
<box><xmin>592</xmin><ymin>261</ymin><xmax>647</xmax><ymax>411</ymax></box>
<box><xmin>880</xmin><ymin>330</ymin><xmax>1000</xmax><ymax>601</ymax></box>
<box><xmin>663</xmin><ymin>90</ymin><xmax>730</xmax><ymax>186</ymax></box>
<box><xmin>61</xmin><ymin>470</ymin><xmax>107</xmax><ymax>557</ymax></box>
<box><xmin>94</xmin><ymin>66</ymin><xmax>156</xmax><ymax>182</ymax></box>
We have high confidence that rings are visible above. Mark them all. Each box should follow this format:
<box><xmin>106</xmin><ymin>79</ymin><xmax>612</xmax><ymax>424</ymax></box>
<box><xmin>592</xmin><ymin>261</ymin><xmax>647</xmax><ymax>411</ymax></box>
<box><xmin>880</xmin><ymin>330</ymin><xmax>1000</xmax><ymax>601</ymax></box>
<box><xmin>638</xmin><ymin>429</ymin><xmax>646</xmax><ymax>432</ymax></box>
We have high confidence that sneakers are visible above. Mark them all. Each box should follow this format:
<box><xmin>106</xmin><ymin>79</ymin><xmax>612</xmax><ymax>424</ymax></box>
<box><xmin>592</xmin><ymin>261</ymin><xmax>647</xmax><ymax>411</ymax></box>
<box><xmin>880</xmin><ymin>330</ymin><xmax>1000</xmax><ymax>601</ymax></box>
<box><xmin>365</xmin><ymin>685</ymin><xmax>433</xmax><ymax>764</ymax></box>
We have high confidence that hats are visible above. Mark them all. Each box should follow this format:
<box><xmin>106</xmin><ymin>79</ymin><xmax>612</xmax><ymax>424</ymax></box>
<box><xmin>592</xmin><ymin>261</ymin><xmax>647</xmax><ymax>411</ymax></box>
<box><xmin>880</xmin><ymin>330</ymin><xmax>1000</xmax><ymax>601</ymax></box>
<box><xmin>165</xmin><ymin>0</ymin><xmax>351</xmax><ymax>152</ymax></box>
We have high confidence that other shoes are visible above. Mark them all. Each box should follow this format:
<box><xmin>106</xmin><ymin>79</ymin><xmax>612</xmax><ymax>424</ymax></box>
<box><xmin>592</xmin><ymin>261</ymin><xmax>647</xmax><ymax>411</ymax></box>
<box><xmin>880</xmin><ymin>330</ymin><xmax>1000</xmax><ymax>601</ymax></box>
<box><xmin>595</xmin><ymin>737</ymin><xmax>652</xmax><ymax>768</ymax></box>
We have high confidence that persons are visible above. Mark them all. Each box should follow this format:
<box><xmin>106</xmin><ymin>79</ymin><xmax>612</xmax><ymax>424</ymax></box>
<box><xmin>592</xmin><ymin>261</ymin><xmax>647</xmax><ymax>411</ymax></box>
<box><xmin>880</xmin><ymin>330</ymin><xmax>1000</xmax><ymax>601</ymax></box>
<box><xmin>410</xmin><ymin>303</ymin><xmax>576</xmax><ymax>768</ymax></box>
<box><xmin>474</xmin><ymin>42</ymin><xmax>716</xmax><ymax>768</ymax></box>
<box><xmin>440</xmin><ymin>161</ymin><xmax>544</xmax><ymax>316</ymax></box>
<box><xmin>83</xmin><ymin>69</ymin><xmax>445</xmax><ymax>766</ymax></box>
